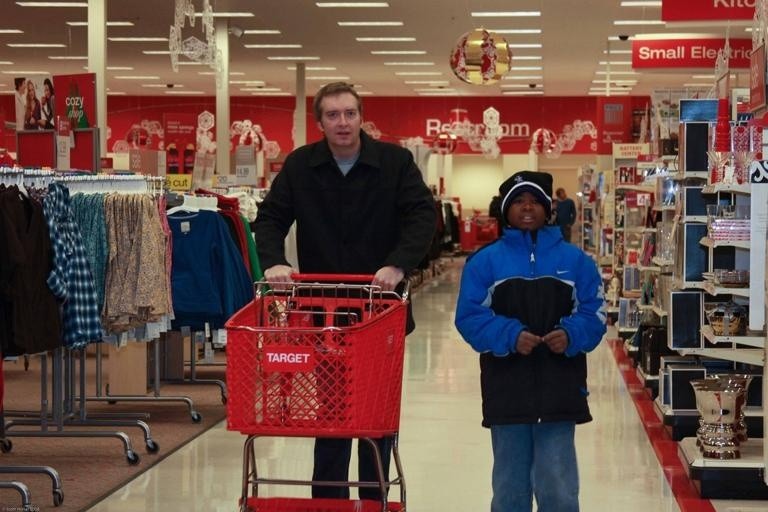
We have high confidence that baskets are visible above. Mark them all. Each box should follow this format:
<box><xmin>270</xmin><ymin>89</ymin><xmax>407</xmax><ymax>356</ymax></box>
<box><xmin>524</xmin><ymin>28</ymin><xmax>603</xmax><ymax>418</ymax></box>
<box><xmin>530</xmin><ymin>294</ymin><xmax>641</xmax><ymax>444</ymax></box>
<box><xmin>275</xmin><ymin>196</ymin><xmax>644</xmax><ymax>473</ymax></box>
<box><xmin>226</xmin><ymin>296</ymin><xmax>408</xmax><ymax>439</ymax></box>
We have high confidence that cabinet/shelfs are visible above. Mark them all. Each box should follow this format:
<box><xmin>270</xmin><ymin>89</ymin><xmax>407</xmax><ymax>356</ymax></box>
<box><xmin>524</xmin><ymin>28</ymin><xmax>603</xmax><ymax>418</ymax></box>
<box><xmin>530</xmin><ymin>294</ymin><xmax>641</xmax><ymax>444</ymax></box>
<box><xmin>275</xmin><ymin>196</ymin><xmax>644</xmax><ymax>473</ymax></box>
<box><xmin>577</xmin><ymin>170</ymin><xmax>767</xmax><ymax>500</ymax></box>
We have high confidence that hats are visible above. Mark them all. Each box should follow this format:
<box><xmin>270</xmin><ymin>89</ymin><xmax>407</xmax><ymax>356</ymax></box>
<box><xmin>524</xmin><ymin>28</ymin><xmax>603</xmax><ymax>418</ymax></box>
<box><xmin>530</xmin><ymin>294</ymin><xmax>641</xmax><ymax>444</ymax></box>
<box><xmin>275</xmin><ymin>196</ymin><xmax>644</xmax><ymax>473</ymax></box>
<box><xmin>499</xmin><ymin>171</ymin><xmax>552</xmax><ymax>223</ymax></box>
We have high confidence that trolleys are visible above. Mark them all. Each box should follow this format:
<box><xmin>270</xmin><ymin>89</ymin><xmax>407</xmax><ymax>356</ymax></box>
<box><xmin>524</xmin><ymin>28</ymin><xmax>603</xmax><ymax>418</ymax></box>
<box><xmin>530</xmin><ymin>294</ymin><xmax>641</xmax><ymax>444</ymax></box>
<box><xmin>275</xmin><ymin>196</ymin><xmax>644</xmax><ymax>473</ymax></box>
<box><xmin>223</xmin><ymin>274</ymin><xmax>411</xmax><ymax>512</ymax></box>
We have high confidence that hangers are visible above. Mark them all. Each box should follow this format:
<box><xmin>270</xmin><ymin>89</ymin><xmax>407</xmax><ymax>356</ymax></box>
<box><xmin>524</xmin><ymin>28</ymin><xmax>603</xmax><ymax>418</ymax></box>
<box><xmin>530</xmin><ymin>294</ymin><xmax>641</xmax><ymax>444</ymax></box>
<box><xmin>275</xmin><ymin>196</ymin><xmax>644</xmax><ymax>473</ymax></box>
<box><xmin>167</xmin><ymin>185</ymin><xmax>268</xmax><ymax>218</ymax></box>
<box><xmin>0</xmin><ymin>164</ymin><xmax>168</xmax><ymax>205</ymax></box>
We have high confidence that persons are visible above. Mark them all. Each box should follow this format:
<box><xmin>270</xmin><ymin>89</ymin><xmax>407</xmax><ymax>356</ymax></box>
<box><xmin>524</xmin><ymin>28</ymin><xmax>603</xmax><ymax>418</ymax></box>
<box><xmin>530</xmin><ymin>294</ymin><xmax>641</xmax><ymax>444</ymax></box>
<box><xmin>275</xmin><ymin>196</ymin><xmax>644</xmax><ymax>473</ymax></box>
<box><xmin>15</xmin><ymin>79</ymin><xmax>26</xmax><ymax>132</ymax></box>
<box><xmin>551</xmin><ymin>199</ymin><xmax>559</xmax><ymax>224</ymax></box>
<box><xmin>555</xmin><ymin>188</ymin><xmax>577</xmax><ymax>243</ymax></box>
<box><xmin>25</xmin><ymin>81</ymin><xmax>41</xmax><ymax>130</ymax></box>
<box><xmin>37</xmin><ymin>79</ymin><xmax>55</xmax><ymax>129</ymax></box>
<box><xmin>254</xmin><ymin>79</ymin><xmax>445</xmax><ymax>499</ymax></box>
<box><xmin>455</xmin><ymin>172</ymin><xmax>606</xmax><ymax>512</ymax></box>
